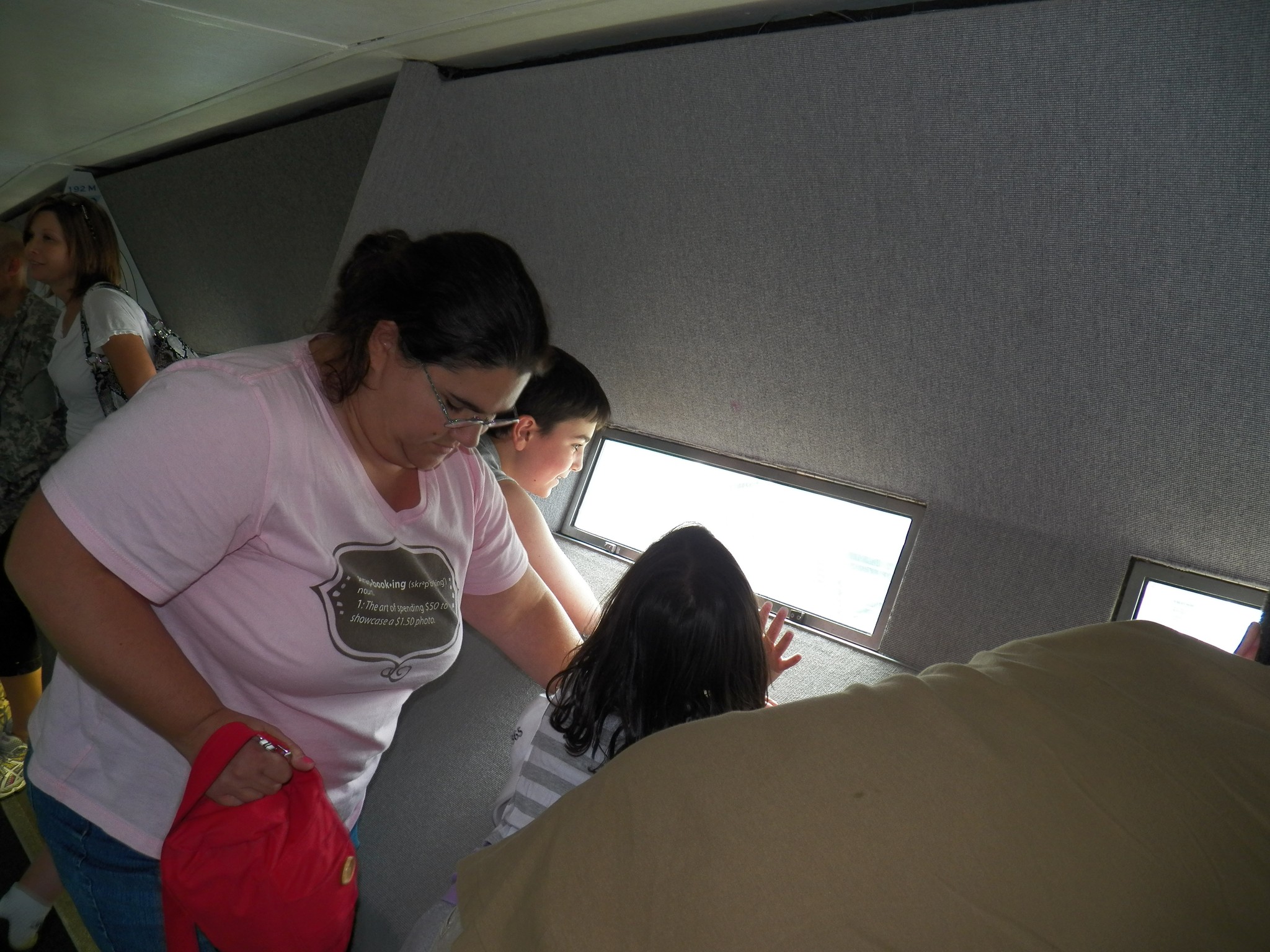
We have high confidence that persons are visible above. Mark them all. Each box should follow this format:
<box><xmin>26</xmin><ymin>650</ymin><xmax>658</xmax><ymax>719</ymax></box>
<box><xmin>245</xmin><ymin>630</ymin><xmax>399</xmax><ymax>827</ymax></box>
<box><xmin>0</xmin><ymin>216</ymin><xmax>63</xmax><ymax>800</ymax></box>
<box><xmin>474</xmin><ymin>345</ymin><xmax>611</xmax><ymax>638</ymax></box>
<box><xmin>487</xmin><ymin>524</ymin><xmax>802</xmax><ymax>845</ymax></box>
<box><xmin>0</xmin><ymin>226</ymin><xmax>582</xmax><ymax>952</ymax></box>
<box><xmin>23</xmin><ymin>194</ymin><xmax>157</xmax><ymax>454</ymax></box>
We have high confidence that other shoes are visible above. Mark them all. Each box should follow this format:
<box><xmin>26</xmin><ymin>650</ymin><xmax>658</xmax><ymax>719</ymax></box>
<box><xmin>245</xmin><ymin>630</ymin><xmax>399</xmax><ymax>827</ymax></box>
<box><xmin>0</xmin><ymin>916</ymin><xmax>51</xmax><ymax>952</ymax></box>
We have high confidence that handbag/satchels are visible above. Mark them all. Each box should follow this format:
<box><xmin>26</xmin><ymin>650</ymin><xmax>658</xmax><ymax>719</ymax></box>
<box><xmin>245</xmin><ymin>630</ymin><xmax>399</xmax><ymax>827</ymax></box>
<box><xmin>80</xmin><ymin>284</ymin><xmax>200</xmax><ymax>417</ymax></box>
<box><xmin>161</xmin><ymin>721</ymin><xmax>357</xmax><ymax>952</ymax></box>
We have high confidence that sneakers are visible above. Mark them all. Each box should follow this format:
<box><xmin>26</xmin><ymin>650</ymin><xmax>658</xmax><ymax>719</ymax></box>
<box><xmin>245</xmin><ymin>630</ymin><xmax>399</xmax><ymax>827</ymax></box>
<box><xmin>0</xmin><ymin>683</ymin><xmax>12</xmax><ymax>735</ymax></box>
<box><xmin>0</xmin><ymin>717</ymin><xmax>28</xmax><ymax>800</ymax></box>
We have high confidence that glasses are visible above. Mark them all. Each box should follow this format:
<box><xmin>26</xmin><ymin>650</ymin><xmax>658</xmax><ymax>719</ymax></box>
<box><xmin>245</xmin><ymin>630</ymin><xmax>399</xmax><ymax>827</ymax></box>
<box><xmin>416</xmin><ymin>354</ymin><xmax>520</xmax><ymax>428</ymax></box>
